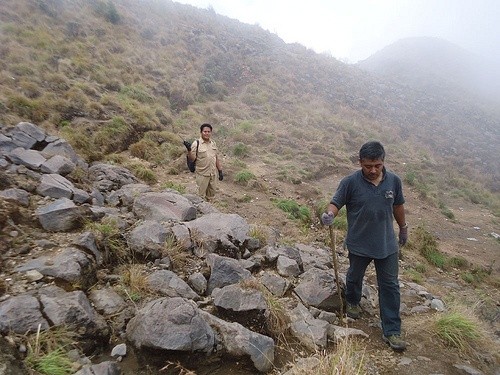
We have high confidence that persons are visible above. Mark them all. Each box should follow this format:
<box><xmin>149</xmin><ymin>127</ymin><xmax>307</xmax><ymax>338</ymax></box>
<box><xmin>321</xmin><ymin>140</ymin><xmax>409</xmax><ymax>350</ymax></box>
<box><xmin>184</xmin><ymin>123</ymin><xmax>223</xmax><ymax>202</ymax></box>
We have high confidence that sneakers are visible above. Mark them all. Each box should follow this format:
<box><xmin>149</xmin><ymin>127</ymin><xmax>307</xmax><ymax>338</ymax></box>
<box><xmin>382</xmin><ymin>333</ymin><xmax>406</xmax><ymax>351</ymax></box>
<box><xmin>346</xmin><ymin>301</ymin><xmax>360</xmax><ymax>318</ymax></box>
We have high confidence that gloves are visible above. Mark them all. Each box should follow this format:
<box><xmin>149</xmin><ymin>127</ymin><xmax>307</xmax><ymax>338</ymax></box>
<box><xmin>184</xmin><ymin>141</ymin><xmax>191</xmax><ymax>151</ymax></box>
<box><xmin>219</xmin><ymin>170</ymin><xmax>223</xmax><ymax>181</ymax></box>
<box><xmin>321</xmin><ymin>211</ymin><xmax>334</xmax><ymax>226</ymax></box>
<box><xmin>398</xmin><ymin>223</ymin><xmax>408</xmax><ymax>247</ymax></box>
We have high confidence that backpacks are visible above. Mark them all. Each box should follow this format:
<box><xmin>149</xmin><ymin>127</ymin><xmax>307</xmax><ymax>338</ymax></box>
<box><xmin>187</xmin><ymin>140</ymin><xmax>199</xmax><ymax>173</ymax></box>
